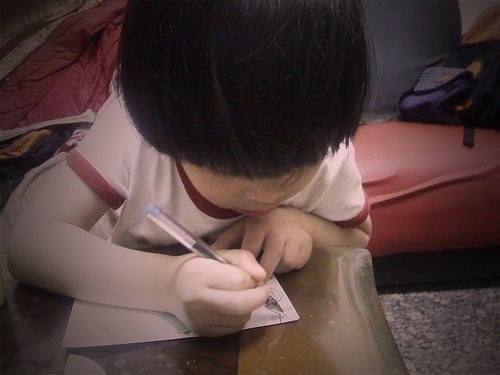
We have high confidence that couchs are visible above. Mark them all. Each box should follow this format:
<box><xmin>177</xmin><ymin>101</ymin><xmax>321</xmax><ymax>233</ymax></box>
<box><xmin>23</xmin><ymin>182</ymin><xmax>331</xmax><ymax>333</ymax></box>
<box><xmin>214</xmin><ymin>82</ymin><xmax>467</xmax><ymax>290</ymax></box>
<box><xmin>0</xmin><ymin>0</ymin><xmax>500</xmax><ymax>259</ymax></box>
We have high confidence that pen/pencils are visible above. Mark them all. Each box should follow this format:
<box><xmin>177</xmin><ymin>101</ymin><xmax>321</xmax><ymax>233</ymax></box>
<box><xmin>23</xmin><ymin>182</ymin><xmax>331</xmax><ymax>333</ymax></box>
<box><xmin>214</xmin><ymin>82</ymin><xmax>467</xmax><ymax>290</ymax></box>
<box><xmin>143</xmin><ymin>202</ymin><xmax>286</xmax><ymax>317</ymax></box>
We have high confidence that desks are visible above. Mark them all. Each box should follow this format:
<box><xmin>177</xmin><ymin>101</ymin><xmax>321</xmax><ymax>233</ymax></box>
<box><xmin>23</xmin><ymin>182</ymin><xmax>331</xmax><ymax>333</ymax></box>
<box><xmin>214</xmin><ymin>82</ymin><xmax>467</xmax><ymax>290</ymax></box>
<box><xmin>0</xmin><ymin>236</ymin><xmax>409</xmax><ymax>375</ymax></box>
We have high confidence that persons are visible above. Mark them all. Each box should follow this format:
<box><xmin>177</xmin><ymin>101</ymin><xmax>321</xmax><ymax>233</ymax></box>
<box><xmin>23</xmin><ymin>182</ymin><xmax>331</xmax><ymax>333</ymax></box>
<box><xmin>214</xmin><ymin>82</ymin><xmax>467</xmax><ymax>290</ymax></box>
<box><xmin>8</xmin><ymin>0</ymin><xmax>374</xmax><ymax>339</ymax></box>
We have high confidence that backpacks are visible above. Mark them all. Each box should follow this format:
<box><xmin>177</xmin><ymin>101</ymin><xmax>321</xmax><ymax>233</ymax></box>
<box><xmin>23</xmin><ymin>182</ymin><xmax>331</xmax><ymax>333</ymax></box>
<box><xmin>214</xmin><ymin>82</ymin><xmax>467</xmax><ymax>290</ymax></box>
<box><xmin>400</xmin><ymin>67</ymin><xmax>476</xmax><ymax>121</ymax></box>
